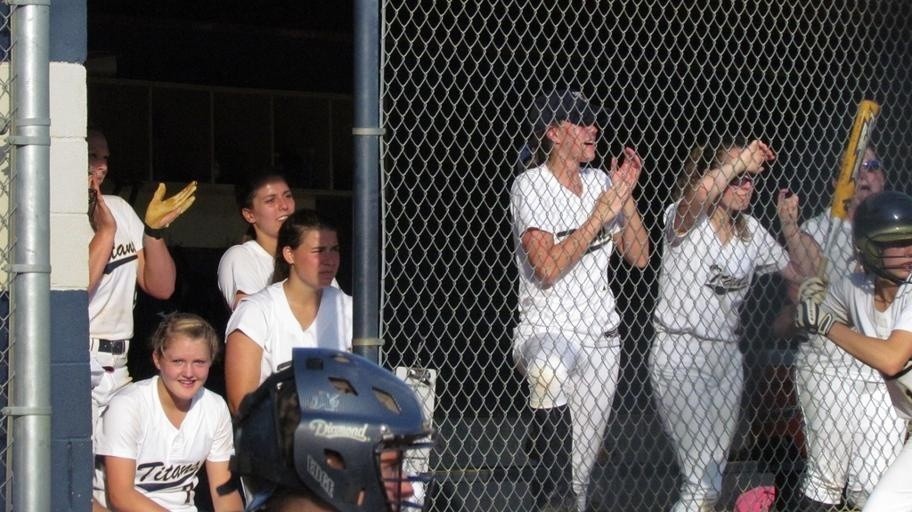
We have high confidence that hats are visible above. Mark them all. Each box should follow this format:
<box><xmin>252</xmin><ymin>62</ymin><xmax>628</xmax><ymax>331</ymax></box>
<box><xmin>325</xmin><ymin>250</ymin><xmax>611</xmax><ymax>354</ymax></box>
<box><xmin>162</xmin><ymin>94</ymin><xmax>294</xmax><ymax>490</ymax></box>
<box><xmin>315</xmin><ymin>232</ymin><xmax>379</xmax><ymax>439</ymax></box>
<box><xmin>531</xmin><ymin>90</ymin><xmax>612</xmax><ymax>127</ymax></box>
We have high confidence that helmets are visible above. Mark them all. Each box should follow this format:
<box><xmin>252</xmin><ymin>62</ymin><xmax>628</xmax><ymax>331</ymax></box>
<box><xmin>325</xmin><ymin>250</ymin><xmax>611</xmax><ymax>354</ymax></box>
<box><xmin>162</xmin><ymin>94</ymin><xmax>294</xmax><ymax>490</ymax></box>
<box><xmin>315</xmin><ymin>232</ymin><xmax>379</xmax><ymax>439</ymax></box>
<box><xmin>852</xmin><ymin>190</ymin><xmax>912</xmax><ymax>284</ymax></box>
<box><xmin>228</xmin><ymin>347</ymin><xmax>434</xmax><ymax>512</ymax></box>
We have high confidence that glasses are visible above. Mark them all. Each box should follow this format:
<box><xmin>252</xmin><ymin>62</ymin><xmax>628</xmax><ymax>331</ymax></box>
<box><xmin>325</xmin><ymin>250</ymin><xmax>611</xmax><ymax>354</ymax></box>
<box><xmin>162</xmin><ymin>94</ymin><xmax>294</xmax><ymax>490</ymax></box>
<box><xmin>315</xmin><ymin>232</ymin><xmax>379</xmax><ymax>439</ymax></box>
<box><xmin>861</xmin><ymin>159</ymin><xmax>882</xmax><ymax>174</ymax></box>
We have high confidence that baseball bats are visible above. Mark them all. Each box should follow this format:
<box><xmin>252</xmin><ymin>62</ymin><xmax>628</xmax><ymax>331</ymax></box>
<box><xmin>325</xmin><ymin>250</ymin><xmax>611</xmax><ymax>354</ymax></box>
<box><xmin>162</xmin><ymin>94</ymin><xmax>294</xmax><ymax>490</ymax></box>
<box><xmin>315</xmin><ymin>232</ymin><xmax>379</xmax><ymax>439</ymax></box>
<box><xmin>817</xmin><ymin>100</ymin><xmax>879</xmax><ymax>278</ymax></box>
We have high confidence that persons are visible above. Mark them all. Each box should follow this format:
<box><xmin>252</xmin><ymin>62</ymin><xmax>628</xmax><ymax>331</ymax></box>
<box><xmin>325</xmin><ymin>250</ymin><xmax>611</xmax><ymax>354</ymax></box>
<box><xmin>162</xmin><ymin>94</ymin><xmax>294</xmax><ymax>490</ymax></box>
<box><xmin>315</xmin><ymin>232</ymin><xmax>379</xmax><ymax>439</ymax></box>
<box><xmin>87</xmin><ymin>128</ymin><xmax>199</xmax><ymax>512</ymax></box>
<box><xmin>224</xmin><ymin>207</ymin><xmax>353</xmax><ymax>413</ymax></box>
<box><xmin>96</xmin><ymin>312</ymin><xmax>246</xmax><ymax>512</ymax></box>
<box><xmin>510</xmin><ymin>90</ymin><xmax>649</xmax><ymax>512</ymax></box>
<box><xmin>229</xmin><ymin>346</ymin><xmax>431</xmax><ymax>511</ymax></box>
<box><xmin>647</xmin><ymin>139</ymin><xmax>815</xmax><ymax>511</ymax></box>
<box><xmin>219</xmin><ymin>168</ymin><xmax>342</xmax><ymax>308</ymax></box>
<box><xmin>786</xmin><ymin>143</ymin><xmax>887</xmax><ymax>512</ymax></box>
<box><xmin>771</xmin><ymin>190</ymin><xmax>911</xmax><ymax>512</ymax></box>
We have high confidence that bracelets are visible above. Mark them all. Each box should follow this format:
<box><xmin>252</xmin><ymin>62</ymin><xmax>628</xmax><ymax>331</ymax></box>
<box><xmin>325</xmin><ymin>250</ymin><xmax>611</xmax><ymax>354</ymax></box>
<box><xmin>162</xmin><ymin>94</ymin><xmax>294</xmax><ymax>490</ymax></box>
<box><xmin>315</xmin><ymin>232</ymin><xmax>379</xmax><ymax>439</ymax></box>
<box><xmin>143</xmin><ymin>223</ymin><xmax>165</xmax><ymax>240</ymax></box>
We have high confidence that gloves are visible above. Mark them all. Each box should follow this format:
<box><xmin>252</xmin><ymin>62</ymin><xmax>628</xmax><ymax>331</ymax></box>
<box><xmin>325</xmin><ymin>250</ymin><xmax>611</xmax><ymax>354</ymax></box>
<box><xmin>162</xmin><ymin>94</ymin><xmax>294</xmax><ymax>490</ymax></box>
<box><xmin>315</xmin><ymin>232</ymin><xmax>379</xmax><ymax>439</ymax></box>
<box><xmin>793</xmin><ymin>277</ymin><xmax>836</xmax><ymax>336</ymax></box>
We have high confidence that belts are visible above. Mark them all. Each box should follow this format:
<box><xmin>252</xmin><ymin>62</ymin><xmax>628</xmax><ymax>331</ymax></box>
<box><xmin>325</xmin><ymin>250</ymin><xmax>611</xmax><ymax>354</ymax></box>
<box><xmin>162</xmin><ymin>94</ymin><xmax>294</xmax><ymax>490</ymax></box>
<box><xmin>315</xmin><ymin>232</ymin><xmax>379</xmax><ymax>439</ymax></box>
<box><xmin>99</xmin><ymin>339</ymin><xmax>126</xmax><ymax>356</ymax></box>
